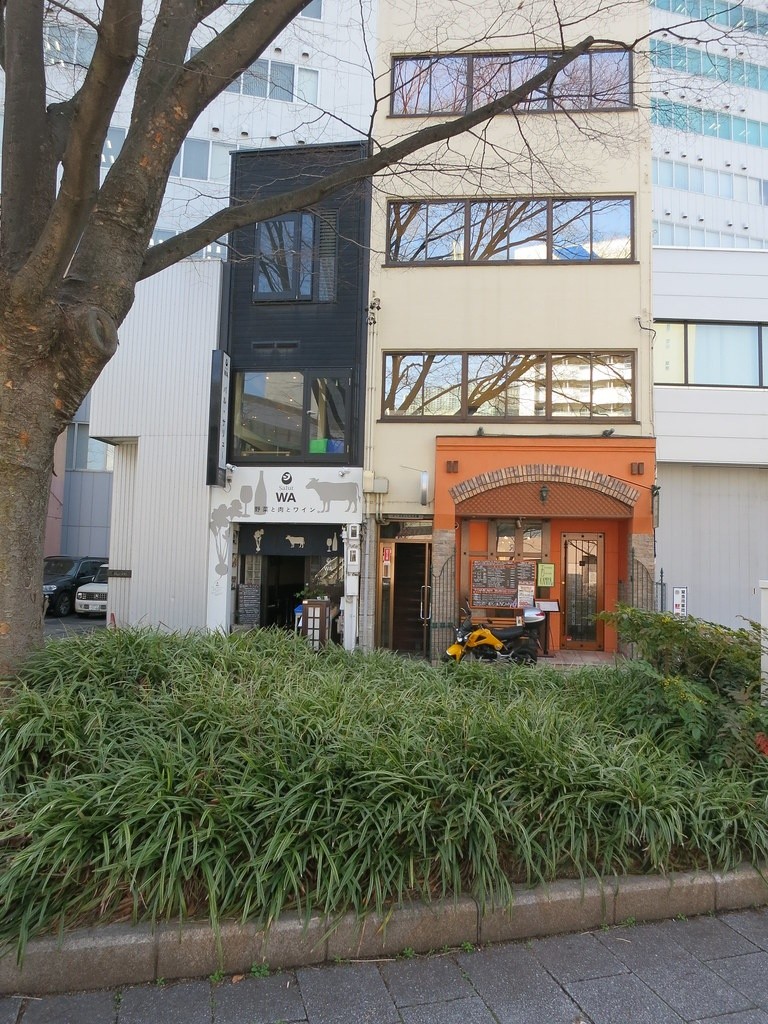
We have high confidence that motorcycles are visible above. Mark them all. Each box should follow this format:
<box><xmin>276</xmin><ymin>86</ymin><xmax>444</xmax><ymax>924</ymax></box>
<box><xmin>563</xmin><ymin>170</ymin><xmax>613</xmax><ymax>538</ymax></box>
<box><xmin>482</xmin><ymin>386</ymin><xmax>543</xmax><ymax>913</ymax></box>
<box><xmin>442</xmin><ymin>595</ymin><xmax>547</xmax><ymax>675</ymax></box>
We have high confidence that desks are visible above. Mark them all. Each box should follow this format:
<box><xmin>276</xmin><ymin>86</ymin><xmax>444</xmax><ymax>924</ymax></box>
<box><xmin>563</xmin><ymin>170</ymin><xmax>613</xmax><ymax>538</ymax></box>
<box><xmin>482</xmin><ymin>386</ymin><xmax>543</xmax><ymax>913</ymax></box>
<box><xmin>241</xmin><ymin>451</ymin><xmax>291</xmax><ymax>456</ymax></box>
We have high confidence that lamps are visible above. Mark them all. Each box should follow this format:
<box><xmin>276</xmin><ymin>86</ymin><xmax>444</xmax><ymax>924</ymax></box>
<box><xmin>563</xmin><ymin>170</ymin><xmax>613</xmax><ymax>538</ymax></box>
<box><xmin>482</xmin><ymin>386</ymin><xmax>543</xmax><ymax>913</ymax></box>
<box><xmin>420</xmin><ymin>471</ymin><xmax>428</xmax><ymax>506</ymax></box>
<box><xmin>539</xmin><ymin>486</ymin><xmax>548</xmax><ymax>502</ymax></box>
<box><xmin>516</xmin><ymin>517</ymin><xmax>526</xmax><ymax>528</ymax></box>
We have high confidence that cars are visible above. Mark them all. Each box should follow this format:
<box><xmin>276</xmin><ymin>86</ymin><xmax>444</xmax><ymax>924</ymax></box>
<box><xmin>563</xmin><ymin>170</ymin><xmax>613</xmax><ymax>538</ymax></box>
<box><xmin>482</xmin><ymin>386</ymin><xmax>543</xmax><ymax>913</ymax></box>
<box><xmin>74</xmin><ymin>564</ymin><xmax>110</xmax><ymax>616</ymax></box>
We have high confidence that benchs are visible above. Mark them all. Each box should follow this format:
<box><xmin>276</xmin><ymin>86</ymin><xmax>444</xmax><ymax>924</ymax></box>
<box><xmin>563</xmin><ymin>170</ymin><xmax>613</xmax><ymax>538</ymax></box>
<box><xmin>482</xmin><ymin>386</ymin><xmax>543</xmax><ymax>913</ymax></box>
<box><xmin>461</xmin><ymin>617</ymin><xmax>529</xmax><ymax>647</ymax></box>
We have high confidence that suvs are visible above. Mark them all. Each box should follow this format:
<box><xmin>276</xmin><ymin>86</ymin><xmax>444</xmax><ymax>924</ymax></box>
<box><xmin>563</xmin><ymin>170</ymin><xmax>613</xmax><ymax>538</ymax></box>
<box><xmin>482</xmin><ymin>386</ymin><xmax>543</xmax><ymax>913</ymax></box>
<box><xmin>40</xmin><ymin>556</ymin><xmax>110</xmax><ymax>618</ymax></box>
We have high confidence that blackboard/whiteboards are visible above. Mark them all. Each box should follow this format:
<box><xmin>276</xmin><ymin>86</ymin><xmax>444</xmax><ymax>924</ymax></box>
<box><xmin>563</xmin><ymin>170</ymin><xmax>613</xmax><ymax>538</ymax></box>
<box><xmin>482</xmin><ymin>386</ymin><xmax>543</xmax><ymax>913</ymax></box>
<box><xmin>470</xmin><ymin>559</ymin><xmax>537</xmax><ymax>610</ymax></box>
